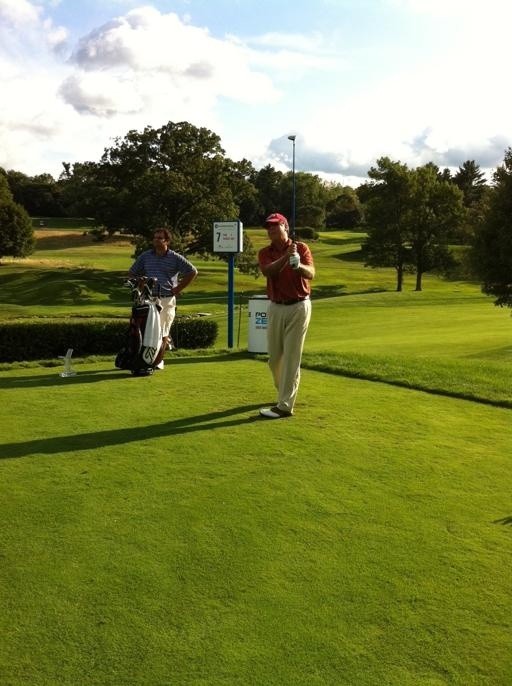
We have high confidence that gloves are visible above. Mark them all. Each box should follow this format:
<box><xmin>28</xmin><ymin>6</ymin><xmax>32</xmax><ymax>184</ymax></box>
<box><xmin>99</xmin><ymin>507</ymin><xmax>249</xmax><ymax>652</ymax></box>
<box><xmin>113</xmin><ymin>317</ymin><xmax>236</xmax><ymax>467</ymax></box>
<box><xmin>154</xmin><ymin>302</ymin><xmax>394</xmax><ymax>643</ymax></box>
<box><xmin>290</xmin><ymin>254</ymin><xmax>300</xmax><ymax>269</ymax></box>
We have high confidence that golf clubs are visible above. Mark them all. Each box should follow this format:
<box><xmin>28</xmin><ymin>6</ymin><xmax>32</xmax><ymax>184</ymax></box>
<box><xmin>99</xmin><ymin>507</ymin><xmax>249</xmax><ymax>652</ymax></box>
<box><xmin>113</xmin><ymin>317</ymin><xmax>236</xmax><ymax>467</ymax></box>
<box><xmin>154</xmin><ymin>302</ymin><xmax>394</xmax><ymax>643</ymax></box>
<box><xmin>284</xmin><ymin>134</ymin><xmax>297</xmax><ymax>269</ymax></box>
<box><xmin>128</xmin><ymin>277</ymin><xmax>159</xmax><ymax>304</ymax></box>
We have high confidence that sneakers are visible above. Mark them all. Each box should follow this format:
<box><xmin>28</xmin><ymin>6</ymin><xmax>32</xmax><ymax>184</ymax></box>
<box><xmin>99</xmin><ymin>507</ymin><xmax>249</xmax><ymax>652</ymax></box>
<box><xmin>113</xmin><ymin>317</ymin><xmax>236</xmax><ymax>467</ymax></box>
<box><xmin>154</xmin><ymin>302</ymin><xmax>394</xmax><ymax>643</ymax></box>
<box><xmin>156</xmin><ymin>360</ymin><xmax>163</xmax><ymax>369</ymax></box>
<box><xmin>260</xmin><ymin>406</ymin><xmax>293</xmax><ymax>417</ymax></box>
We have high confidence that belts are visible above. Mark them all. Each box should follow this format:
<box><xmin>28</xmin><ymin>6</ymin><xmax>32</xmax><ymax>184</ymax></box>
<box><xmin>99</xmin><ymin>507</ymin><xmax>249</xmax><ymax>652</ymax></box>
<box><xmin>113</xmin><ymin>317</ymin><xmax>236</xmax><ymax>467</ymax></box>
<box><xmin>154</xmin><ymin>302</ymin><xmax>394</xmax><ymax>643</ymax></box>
<box><xmin>274</xmin><ymin>296</ymin><xmax>309</xmax><ymax>305</ymax></box>
<box><xmin>161</xmin><ymin>294</ymin><xmax>173</xmax><ymax>297</ymax></box>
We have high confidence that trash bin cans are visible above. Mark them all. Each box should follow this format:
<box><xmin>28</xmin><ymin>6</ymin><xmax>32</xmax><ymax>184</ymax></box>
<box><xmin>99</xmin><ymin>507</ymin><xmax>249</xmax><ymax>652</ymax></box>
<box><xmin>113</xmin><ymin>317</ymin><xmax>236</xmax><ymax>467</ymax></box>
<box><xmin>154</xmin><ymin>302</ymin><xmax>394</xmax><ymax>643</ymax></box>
<box><xmin>247</xmin><ymin>295</ymin><xmax>274</xmax><ymax>353</ymax></box>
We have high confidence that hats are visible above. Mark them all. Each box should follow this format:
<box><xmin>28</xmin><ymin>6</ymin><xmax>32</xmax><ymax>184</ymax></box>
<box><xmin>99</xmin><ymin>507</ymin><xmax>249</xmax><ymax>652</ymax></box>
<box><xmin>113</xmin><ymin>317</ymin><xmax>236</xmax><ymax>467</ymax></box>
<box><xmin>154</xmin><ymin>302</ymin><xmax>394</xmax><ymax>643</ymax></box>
<box><xmin>262</xmin><ymin>214</ymin><xmax>287</xmax><ymax>222</ymax></box>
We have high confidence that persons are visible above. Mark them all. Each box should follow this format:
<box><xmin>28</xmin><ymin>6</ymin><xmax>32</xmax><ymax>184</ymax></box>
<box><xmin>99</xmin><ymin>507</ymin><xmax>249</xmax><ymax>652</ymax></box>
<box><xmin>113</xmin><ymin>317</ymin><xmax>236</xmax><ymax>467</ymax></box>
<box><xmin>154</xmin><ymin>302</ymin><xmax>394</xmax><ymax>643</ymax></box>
<box><xmin>255</xmin><ymin>212</ymin><xmax>317</xmax><ymax>420</ymax></box>
<box><xmin>126</xmin><ymin>222</ymin><xmax>199</xmax><ymax>373</ymax></box>
